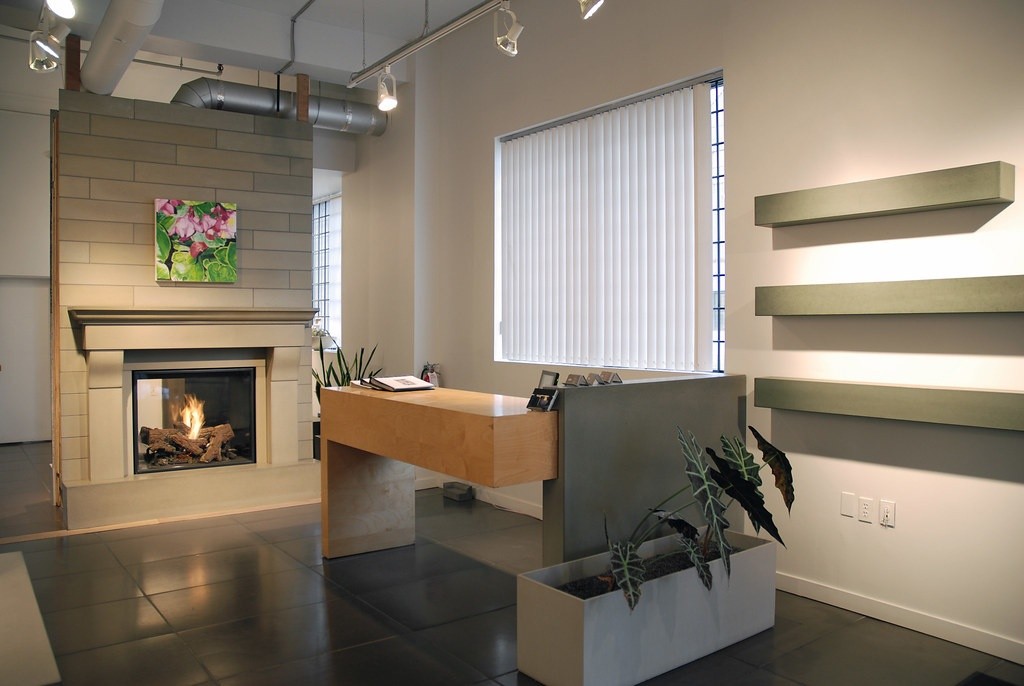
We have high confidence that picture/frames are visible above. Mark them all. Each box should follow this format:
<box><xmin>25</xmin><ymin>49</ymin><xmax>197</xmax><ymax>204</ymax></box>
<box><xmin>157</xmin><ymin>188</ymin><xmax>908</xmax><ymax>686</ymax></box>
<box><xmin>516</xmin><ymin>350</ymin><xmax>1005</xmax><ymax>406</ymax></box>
<box><xmin>154</xmin><ymin>198</ymin><xmax>238</xmax><ymax>283</ymax></box>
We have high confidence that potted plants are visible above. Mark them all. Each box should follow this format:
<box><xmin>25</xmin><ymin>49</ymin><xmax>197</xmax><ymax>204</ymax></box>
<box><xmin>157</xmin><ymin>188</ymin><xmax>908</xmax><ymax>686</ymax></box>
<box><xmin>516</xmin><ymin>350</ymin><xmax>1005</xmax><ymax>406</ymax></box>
<box><xmin>516</xmin><ymin>425</ymin><xmax>779</xmax><ymax>686</ymax></box>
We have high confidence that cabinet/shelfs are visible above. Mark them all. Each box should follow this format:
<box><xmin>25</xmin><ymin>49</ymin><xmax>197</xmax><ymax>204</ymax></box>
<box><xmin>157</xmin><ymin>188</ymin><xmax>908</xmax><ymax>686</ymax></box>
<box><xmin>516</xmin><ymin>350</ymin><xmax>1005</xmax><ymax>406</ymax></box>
<box><xmin>753</xmin><ymin>160</ymin><xmax>1024</xmax><ymax>430</ymax></box>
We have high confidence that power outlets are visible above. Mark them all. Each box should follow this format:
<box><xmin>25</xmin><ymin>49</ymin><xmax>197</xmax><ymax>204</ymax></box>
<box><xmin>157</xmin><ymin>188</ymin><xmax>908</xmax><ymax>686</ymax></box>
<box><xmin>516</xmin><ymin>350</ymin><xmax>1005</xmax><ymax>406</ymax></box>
<box><xmin>840</xmin><ymin>492</ymin><xmax>856</xmax><ymax>518</ymax></box>
<box><xmin>859</xmin><ymin>497</ymin><xmax>875</xmax><ymax>523</ymax></box>
<box><xmin>880</xmin><ymin>500</ymin><xmax>896</xmax><ymax>527</ymax></box>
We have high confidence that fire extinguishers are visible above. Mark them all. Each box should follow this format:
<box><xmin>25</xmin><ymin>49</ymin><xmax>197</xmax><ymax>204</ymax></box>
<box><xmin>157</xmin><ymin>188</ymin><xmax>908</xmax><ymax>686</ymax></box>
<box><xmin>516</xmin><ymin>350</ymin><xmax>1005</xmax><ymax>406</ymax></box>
<box><xmin>421</xmin><ymin>361</ymin><xmax>441</xmax><ymax>386</ymax></box>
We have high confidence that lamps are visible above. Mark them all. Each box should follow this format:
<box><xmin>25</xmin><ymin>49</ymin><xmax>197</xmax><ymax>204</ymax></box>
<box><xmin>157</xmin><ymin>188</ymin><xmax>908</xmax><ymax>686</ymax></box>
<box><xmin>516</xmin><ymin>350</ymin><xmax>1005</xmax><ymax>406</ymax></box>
<box><xmin>577</xmin><ymin>0</ymin><xmax>604</xmax><ymax>21</ymax></box>
<box><xmin>27</xmin><ymin>0</ymin><xmax>77</xmax><ymax>73</ymax></box>
<box><xmin>377</xmin><ymin>63</ymin><xmax>399</xmax><ymax>111</ymax></box>
<box><xmin>493</xmin><ymin>0</ymin><xmax>524</xmax><ymax>58</ymax></box>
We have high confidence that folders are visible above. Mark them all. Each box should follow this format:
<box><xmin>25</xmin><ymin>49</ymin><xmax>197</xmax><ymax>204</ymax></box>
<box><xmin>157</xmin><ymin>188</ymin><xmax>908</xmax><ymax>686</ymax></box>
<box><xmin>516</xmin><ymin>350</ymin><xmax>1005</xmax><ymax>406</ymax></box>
<box><xmin>350</xmin><ymin>374</ymin><xmax>434</xmax><ymax>392</ymax></box>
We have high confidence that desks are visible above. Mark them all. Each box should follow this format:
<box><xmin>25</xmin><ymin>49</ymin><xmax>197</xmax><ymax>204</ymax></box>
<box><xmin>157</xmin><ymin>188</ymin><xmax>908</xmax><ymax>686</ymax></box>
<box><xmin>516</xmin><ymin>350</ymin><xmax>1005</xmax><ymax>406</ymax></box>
<box><xmin>319</xmin><ymin>385</ymin><xmax>558</xmax><ymax>560</ymax></box>
